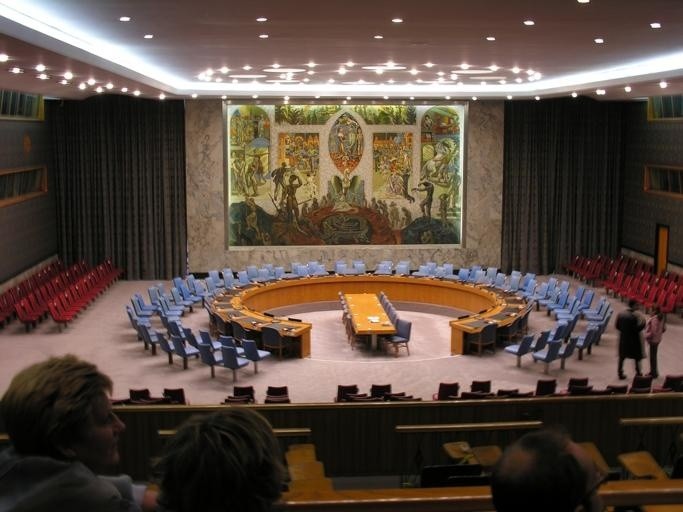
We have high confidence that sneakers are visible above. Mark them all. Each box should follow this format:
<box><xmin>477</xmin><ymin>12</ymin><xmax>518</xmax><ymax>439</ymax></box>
<box><xmin>620</xmin><ymin>371</ymin><xmax>653</xmax><ymax>380</ymax></box>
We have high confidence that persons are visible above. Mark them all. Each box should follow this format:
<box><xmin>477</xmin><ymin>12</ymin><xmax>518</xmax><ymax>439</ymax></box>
<box><xmin>614</xmin><ymin>297</ymin><xmax>647</xmax><ymax>381</ymax></box>
<box><xmin>642</xmin><ymin>304</ymin><xmax>668</xmax><ymax>380</ymax></box>
<box><xmin>230</xmin><ymin>110</ymin><xmax>462</xmax><ymax>244</ymax></box>
<box><xmin>148</xmin><ymin>406</ymin><xmax>291</xmax><ymax>512</ymax></box>
<box><xmin>490</xmin><ymin>425</ymin><xmax>608</xmax><ymax>512</ymax></box>
<box><xmin>0</xmin><ymin>351</ymin><xmax>160</xmax><ymax>512</ymax></box>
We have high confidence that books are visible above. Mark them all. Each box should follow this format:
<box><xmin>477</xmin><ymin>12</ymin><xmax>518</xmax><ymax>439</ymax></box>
<box><xmin>382</xmin><ymin>320</ymin><xmax>391</xmax><ymax>327</ymax></box>
<box><xmin>367</xmin><ymin>315</ymin><xmax>381</xmax><ymax>323</ymax></box>
